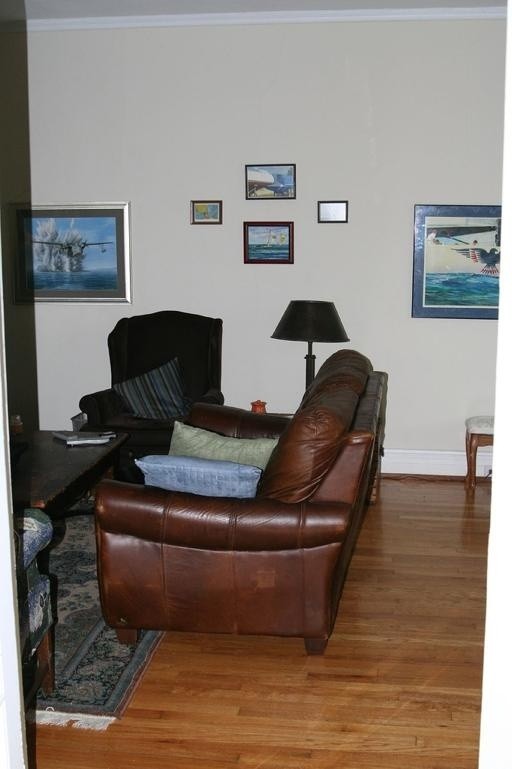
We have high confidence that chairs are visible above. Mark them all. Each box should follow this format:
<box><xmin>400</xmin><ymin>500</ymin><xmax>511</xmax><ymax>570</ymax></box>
<box><xmin>484</xmin><ymin>465</ymin><xmax>492</xmax><ymax>475</ymax></box>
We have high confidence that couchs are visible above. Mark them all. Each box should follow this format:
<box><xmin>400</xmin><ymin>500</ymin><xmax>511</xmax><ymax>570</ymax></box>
<box><xmin>14</xmin><ymin>508</ymin><xmax>53</xmax><ymax>711</ymax></box>
<box><xmin>96</xmin><ymin>349</ymin><xmax>388</xmax><ymax>653</ymax></box>
<box><xmin>79</xmin><ymin>310</ymin><xmax>223</xmax><ymax>483</ymax></box>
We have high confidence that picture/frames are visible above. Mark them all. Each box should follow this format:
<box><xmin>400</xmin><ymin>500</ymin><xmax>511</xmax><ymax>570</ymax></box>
<box><xmin>245</xmin><ymin>164</ymin><xmax>296</xmax><ymax>200</ymax></box>
<box><xmin>15</xmin><ymin>201</ymin><xmax>131</xmax><ymax>304</ymax></box>
<box><xmin>412</xmin><ymin>204</ymin><xmax>502</xmax><ymax>319</ymax></box>
<box><xmin>190</xmin><ymin>200</ymin><xmax>222</xmax><ymax>224</ymax></box>
<box><xmin>242</xmin><ymin>221</ymin><xmax>294</xmax><ymax>264</ymax></box>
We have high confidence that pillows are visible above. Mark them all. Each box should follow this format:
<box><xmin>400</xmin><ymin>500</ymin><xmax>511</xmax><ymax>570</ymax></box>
<box><xmin>133</xmin><ymin>454</ymin><xmax>262</xmax><ymax>498</ymax></box>
<box><xmin>245</xmin><ymin>389</ymin><xmax>359</xmax><ymax>504</ymax></box>
<box><xmin>112</xmin><ymin>358</ymin><xmax>195</xmax><ymax>421</ymax></box>
<box><xmin>277</xmin><ymin>349</ymin><xmax>372</xmax><ymax>438</ymax></box>
<box><xmin>168</xmin><ymin>421</ymin><xmax>278</xmax><ymax>482</ymax></box>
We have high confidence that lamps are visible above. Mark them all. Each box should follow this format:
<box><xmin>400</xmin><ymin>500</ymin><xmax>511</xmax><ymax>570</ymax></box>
<box><xmin>271</xmin><ymin>300</ymin><xmax>350</xmax><ymax>390</ymax></box>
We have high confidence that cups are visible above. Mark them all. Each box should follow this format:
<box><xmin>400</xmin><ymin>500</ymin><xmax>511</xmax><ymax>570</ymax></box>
<box><xmin>251</xmin><ymin>400</ymin><xmax>266</xmax><ymax>414</ymax></box>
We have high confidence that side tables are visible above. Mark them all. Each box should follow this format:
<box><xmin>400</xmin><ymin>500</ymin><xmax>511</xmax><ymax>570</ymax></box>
<box><xmin>265</xmin><ymin>415</ymin><xmax>294</xmax><ymax>419</ymax></box>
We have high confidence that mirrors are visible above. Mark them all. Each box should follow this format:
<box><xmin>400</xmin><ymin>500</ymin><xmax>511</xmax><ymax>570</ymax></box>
<box><xmin>318</xmin><ymin>200</ymin><xmax>348</xmax><ymax>222</ymax></box>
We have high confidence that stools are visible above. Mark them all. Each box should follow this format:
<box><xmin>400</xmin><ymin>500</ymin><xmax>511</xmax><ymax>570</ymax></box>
<box><xmin>465</xmin><ymin>417</ymin><xmax>494</xmax><ymax>489</ymax></box>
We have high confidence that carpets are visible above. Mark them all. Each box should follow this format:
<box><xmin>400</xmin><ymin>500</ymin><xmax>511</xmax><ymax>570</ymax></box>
<box><xmin>25</xmin><ymin>479</ymin><xmax>166</xmax><ymax>733</ymax></box>
